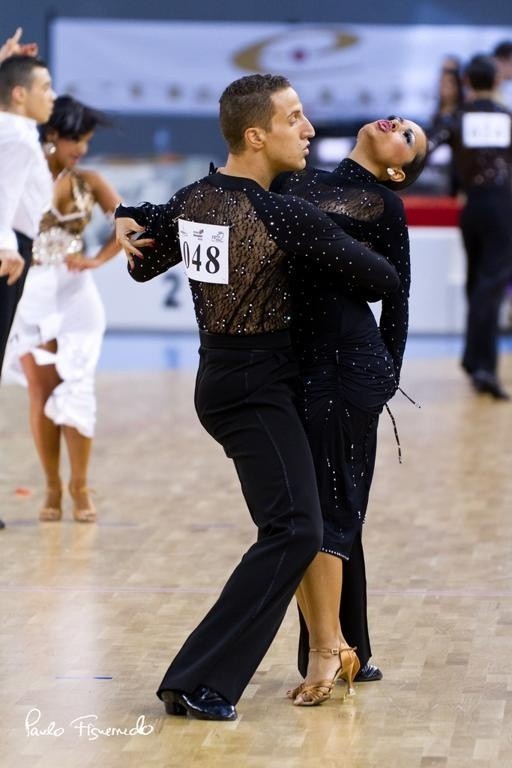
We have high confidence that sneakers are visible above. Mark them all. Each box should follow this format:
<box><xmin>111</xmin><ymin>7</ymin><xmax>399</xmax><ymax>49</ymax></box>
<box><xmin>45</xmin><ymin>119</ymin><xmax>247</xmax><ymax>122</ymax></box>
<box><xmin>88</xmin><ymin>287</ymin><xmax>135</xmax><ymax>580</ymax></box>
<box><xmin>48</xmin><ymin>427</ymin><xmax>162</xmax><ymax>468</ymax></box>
<box><xmin>462</xmin><ymin>359</ymin><xmax>510</xmax><ymax>400</ymax></box>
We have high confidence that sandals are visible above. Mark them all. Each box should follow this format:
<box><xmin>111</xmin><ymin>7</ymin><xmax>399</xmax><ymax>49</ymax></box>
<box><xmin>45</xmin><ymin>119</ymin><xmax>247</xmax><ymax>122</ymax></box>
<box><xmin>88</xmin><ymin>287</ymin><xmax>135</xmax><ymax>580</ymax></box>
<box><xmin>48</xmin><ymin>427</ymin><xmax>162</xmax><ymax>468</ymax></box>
<box><xmin>66</xmin><ymin>482</ymin><xmax>99</xmax><ymax>526</ymax></box>
<box><xmin>37</xmin><ymin>479</ymin><xmax>64</xmax><ymax>523</ymax></box>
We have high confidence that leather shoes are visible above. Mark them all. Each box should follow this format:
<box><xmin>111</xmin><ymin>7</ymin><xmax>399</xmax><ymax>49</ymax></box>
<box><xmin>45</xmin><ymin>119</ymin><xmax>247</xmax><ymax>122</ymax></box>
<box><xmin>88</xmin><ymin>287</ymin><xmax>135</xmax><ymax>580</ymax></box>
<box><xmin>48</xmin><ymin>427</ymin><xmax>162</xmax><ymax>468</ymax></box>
<box><xmin>354</xmin><ymin>665</ymin><xmax>384</xmax><ymax>682</ymax></box>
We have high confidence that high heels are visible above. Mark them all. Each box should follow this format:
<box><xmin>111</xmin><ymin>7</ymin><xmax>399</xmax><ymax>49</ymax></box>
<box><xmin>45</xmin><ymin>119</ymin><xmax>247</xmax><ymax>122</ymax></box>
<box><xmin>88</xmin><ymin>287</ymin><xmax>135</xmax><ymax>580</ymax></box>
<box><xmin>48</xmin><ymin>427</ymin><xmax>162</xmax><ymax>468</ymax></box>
<box><xmin>285</xmin><ymin>640</ymin><xmax>361</xmax><ymax>705</ymax></box>
<box><xmin>161</xmin><ymin>689</ymin><xmax>239</xmax><ymax>723</ymax></box>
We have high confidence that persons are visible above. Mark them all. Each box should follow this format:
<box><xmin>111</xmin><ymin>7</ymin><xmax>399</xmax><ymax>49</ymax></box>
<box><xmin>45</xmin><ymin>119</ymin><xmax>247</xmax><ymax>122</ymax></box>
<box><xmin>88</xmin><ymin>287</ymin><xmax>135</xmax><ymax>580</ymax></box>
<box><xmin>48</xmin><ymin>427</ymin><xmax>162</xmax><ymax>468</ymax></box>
<box><xmin>430</xmin><ymin>71</ymin><xmax>463</xmax><ymax>143</ymax></box>
<box><xmin>492</xmin><ymin>41</ymin><xmax>511</xmax><ymax>84</ymax></box>
<box><xmin>1</xmin><ymin>27</ymin><xmax>55</xmax><ymax>531</ymax></box>
<box><xmin>113</xmin><ymin>113</ymin><xmax>430</xmax><ymax>708</ymax></box>
<box><xmin>416</xmin><ymin>55</ymin><xmax>511</xmax><ymax>402</ymax></box>
<box><xmin>434</xmin><ymin>57</ymin><xmax>464</xmax><ymax>124</ymax></box>
<box><xmin>6</xmin><ymin>95</ymin><xmax>132</xmax><ymax>523</ymax></box>
<box><xmin>127</xmin><ymin>73</ymin><xmax>402</xmax><ymax>722</ymax></box>
